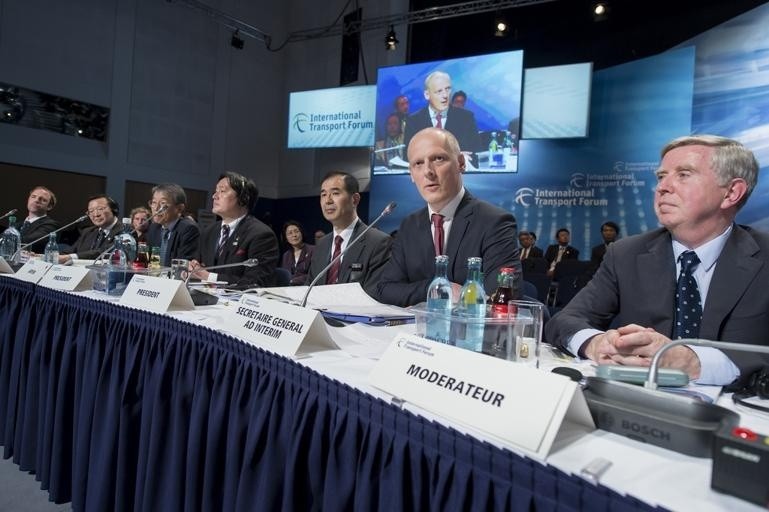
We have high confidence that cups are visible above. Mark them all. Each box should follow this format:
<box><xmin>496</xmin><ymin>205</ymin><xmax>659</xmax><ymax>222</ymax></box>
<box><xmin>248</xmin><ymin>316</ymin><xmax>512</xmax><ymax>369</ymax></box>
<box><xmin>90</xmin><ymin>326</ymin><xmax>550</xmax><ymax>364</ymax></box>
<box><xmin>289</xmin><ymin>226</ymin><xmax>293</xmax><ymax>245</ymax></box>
<box><xmin>170</xmin><ymin>258</ymin><xmax>189</xmax><ymax>286</ymax></box>
<box><xmin>505</xmin><ymin>300</ymin><xmax>544</xmax><ymax>369</ymax></box>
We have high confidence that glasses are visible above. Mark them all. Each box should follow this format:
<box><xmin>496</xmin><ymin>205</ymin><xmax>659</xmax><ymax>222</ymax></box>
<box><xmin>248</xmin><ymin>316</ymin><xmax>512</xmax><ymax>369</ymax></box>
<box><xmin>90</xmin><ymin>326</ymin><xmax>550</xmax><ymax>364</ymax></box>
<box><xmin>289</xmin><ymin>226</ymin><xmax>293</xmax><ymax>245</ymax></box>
<box><xmin>148</xmin><ymin>200</ymin><xmax>174</xmax><ymax>208</ymax></box>
<box><xmin>87</xmin><ymin>206</ymin><xmax>107</xmax><ymax>216</ymax></box>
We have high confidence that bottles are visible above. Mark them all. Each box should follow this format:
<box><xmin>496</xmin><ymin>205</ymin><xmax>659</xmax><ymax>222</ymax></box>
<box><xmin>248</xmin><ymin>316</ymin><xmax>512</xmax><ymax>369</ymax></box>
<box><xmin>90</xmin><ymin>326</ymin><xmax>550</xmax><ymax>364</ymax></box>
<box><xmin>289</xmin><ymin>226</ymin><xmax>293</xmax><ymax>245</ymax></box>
<box><xmin>486</xmin><ymin>129</ymin><xmax>517</xmax><ymax>162</ymax></box>
<box><xmin>0</xmin><ymin>216</ymin><xmax>22</xmax><ymax>267</ymax></box>
<box><xmin>425</xmin><ymin>254</ymin><xmax>519</xmax><ymax>360</ymax></box>
<box><xmin>43</xmin><ymin>232</ymin><xmax>59</xmax><ymax>265</ymax></box>
<box><xmin>107</xmin><ymin>235</ymin><xmax>162</xmax><ymax>296</ymax></box>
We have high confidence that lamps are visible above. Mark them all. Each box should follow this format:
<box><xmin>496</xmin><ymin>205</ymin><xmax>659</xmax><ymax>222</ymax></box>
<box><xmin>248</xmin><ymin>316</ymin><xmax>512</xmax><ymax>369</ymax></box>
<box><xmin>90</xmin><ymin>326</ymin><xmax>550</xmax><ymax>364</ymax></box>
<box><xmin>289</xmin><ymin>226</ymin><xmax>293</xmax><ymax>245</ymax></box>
<box><xmin>384</xmin><ymin>25</ymin><xmax>399</xmax><ymax>46</ymax></box>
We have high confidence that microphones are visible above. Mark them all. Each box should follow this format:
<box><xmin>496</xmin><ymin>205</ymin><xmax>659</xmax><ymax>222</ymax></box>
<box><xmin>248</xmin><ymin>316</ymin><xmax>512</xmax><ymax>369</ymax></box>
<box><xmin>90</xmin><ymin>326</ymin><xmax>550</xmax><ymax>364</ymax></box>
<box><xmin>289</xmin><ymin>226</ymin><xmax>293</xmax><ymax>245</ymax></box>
<box><xmin>0</xmin><ymin>209</ymin><xmax>18</xmax><ymax>219</ymax></box>
<box><xmin>375</xmin><ymin>144</ymin><xmax>407</xmax><ymax>154</ymax></box>
<box><xmin>300</xmin><ymin>201</ymin><xmax>397</xmax><ymax>307</ymax></box>
<box><xmin>10</xmin><ymin>215</ymin><xmax>89</xmax><ymax>261</ymax></box>
<box><xmin>185</xmin><ymin>257</ymin><xmax>259</xmax><ymax>285</ymax></box>
<box><xmin>92</xmin><ymin>204</ymin><xmax>169</xmax><ymax>267</ymax></box>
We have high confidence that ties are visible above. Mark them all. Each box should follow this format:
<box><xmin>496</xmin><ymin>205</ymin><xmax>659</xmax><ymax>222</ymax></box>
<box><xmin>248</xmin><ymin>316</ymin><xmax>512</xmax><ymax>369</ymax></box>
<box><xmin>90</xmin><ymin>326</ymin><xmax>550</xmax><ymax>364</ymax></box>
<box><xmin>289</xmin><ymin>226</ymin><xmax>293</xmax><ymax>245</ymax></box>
<box><xmin>326</xmin><ymin>235</ymin><xmax>344</xmax><ymax>284</ymax></box>
<box><xmin>671</xmin><ymin>252</ymin><xmax>703</xmax><ymax>339</ymax></box>
<box><xmin>217</xmin><ymin>224</ymin><xmax>229</xmax><ymax>258</ymax></box>
<box><xmin>159</xmin><ymin>228</ymin><xmax>170</xmax><ymax>266</ymax></box>
<box><xmin>432</xmin><ymin>214</ymin><xmax>445</xmax><ymax>256</ymax></box>
<box><xmin>522</xmin><ymin>249</ymin><xmax>526</xmax><ymax>259</ymax></box>
<box><xmin>435</xmin><ymin>114</ymin><xmax>442</xmax><ymax>128</ymax></box>
<box><xmin>20</xmin><ymin>221</ymin><xmax>29</xmax><ymax>235</ymax></box>
<box><xmin>95</xmin><ymin>231</ymin><xmax>107</xmax><ymax>250</ymax></box>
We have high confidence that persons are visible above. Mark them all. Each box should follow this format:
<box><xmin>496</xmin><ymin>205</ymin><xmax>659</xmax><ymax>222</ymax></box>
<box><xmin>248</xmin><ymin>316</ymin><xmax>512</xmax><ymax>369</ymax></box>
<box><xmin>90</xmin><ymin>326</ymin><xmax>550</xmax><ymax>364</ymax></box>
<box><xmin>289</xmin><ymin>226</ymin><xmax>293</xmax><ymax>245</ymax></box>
<box><xmin>395</xmin><ymin>94</ymin><xmax>412</xmax><ymax>131</ymax></box>
<box><xmin>281</xmin><ymin>220</ymin><xmax>316</xmax><ymax>283</ymax></box>
<box><xmin>401</xmin><ymin>73</ymin><xmax>479</xmax><ymax>155</ymax></box>
<box><xmin>375</xmin><ymin>113</ymin><xmax>405</xmax><ymax>161</ymax></box>
<box><xmin>303</xmin><ymin>172</ymin><xmax>393</xmax><ymax>286</ymax></box>
<box><xmin>142</xmin><ymin>183</ymin><xmax>197</xmax><ymax>268</ymax></box>
<box><xmin>451</xmin><ymin>90</ymin><xmax>467</xmax><ymax>109</ymax></box>
<box><xmin>517</xmin><ymin>231</ymin><xmax>544</xmax><ymax>301</ymax></box>
<box><xmin>312</xmin><ymin>228</ymin><xmax>329</xmax><ymax>244</ymax></box>
<box><xmin>128</xmin><ymin>208</ymin><xmax>154</xmax><ymax>242</ymax></box>
<box><xmin>363</xmin><ymin>130</ymin><xmax>525</xmax><ymax>305</ymax></box>
<box><xmin>588</xmin><ymin>222</ymin><xmax>621</xmax><ymax>278</ymax></box>
<box><xmin>528</xmin><ymin>232</ymin><xmax>546</xmax><ymax>257</ymax></box>
<box><xmin>11</xmin><ymin>187</ymin><xmax>62</xmax><ymax>256</ymax></box>
<box><xmin>51</xmin><ymin>193</ymin><xmax>122</xmax><ymax>262</ymax></box>
<box><xmin>546</xmin><ymin>133</ymin><xmax>769</xmax><ymax>394</ymax></box>
<box><xmin>180</xmin><ymin>171</ymin><xmax>280</xmax><ymax>293</ymax></box>
<box><xmin>544</xmin><ymin>228</ymin><xmax>580</xmax><ymax>308</ymax></box>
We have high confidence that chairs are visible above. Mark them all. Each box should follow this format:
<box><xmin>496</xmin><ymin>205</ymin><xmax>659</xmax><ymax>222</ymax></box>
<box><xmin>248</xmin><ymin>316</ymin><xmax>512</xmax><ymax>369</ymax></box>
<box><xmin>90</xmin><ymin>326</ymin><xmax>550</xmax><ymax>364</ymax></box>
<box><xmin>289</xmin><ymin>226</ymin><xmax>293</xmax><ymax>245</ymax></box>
<box><xmin>523</xmin><ymin>255</ymin><xmax>601</xmax><ymax>313</ymax></box>
